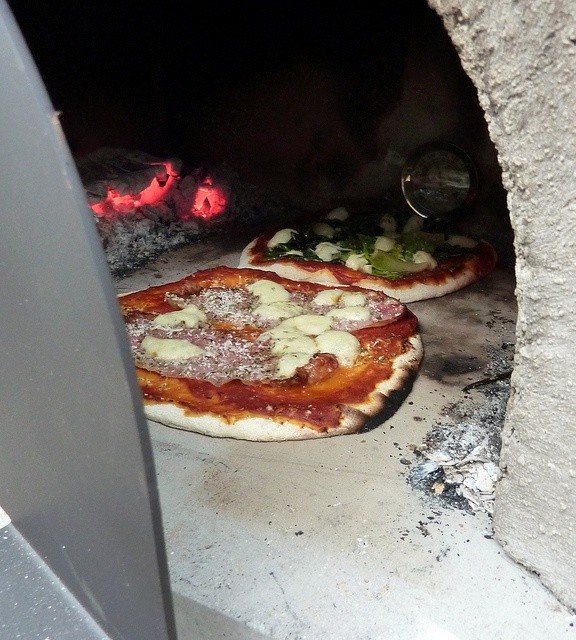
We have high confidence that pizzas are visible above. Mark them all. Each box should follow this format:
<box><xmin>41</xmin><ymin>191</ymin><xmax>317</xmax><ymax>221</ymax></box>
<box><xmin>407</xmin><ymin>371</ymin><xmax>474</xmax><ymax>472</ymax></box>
<box><xmin>240</xmin><ymin>208</ymin><xmax>498</xmax><ymax>303</ymax></box>
<box><xmin>117</xmin><ymin>266</ymin><xmax>424</xmax><ymax>442</ymax></box>
